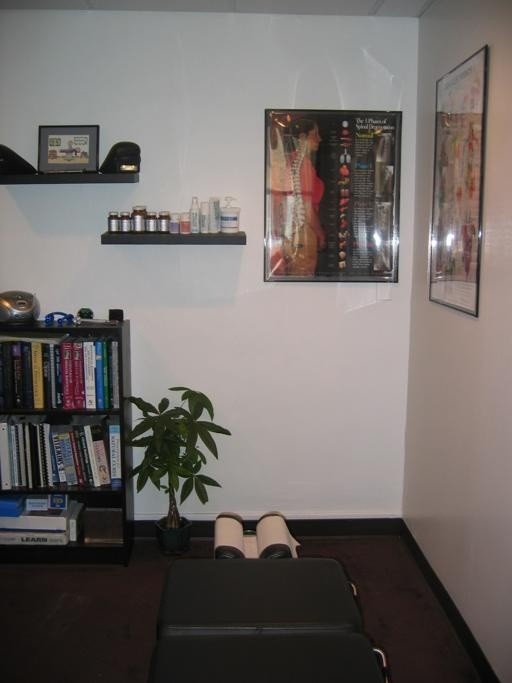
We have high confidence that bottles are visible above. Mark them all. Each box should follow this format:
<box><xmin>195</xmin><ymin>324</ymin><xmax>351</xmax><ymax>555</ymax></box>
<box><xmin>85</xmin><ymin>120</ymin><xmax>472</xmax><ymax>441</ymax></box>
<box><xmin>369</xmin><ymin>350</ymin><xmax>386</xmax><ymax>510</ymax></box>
<box><xmin>145</xmin><ymin>211</ymin><xmax>157</xmax><ymax>232</ymax></box>
<box><xmin>157</xmin><ymin>211</ymin><xmax>170</xmax><ymax>233</ymax></box>
<box><xmin>107</xmin><ymin>211</ymin><xmax>119</xmax><ymax>233</ymax></box>
<box><xmin>119</xmin><ymin>210</ymin><xmax>131</xmax><ymax>233</ymax></box>
<box><xmin>179</xmin><ymin>215</ymin><xmax>190</xmax><ymax>234</ymax></box>
<box><xmin>131</xmin><ymin>206</ymin><xmax>146</xmax><ymax>233</ymax></box>
<box><xmin>169</xmin><ymin>215</ymin><xmax>180</xmax><ymax>234</ymax></box>
<box><xmin>199</xmin><ymin>201</ymin><xmax>209</xmax><ymax>233</ymax></box>
<box><xmin>208</xmin><ymin>197</ymin><xmax>220</xmax><ymax>233</ymax></box>
<box><xmin>189</xmin><ymin>197</ymin><xmax>199</xmax><ymax>233</ymax></box>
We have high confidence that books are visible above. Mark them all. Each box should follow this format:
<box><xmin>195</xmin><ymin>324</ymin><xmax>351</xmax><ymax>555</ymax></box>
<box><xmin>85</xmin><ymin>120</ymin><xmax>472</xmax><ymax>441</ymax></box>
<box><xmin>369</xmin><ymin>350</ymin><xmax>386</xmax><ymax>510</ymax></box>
<box><xmin>0</xmin><ymin>339</ymin><xmax>125</xmax><ymax>548</ymax></box>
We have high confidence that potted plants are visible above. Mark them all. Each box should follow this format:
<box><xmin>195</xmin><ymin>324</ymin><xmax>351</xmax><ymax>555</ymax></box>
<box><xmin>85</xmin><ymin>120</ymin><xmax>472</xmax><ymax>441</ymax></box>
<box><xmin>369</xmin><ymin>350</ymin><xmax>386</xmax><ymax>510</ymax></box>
<box><xmin>119</xmin><ymin>386</ymin><xmax>232</xmax><ymax>556</ymax></box>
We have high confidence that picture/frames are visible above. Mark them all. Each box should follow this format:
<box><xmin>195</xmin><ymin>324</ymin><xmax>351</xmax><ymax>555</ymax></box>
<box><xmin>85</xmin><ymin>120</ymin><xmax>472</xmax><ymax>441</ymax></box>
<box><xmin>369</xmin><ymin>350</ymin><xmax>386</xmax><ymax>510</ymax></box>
<box><xmin>35</xmin><ymin>124</ymin><xmax>100</xmax><ymax>174</ymax></box>
<box><xmin>263</xmin><ymin>108</ymin><xmax>403</xmax><ymax>284</ymax></box>
<box><xmin>426</xmin><ymin>43</ymin><xmax>490</xmax><ymax>318</ymax></box>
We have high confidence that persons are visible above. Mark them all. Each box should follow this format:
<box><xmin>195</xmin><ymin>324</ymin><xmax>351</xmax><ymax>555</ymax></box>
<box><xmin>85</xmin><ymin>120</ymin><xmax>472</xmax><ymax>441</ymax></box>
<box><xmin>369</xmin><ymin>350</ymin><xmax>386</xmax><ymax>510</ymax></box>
<box><xmin>278</xmin><ymin>119</ymin><xmax>329</xmax><ymax>278</ymax></box>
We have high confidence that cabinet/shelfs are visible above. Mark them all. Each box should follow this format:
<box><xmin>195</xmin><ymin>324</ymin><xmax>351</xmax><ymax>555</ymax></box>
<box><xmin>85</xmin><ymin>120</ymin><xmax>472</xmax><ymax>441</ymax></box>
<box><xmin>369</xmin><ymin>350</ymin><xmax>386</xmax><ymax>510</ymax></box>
<box><xmin>0</xmin><ymin>170</ymin><xmax>246</xmax><ymax>247</ymax></box>
<box><xmin>0</xmin><ymin>315</ymin><xmax>135</xmax><ymax>568</ymax></box>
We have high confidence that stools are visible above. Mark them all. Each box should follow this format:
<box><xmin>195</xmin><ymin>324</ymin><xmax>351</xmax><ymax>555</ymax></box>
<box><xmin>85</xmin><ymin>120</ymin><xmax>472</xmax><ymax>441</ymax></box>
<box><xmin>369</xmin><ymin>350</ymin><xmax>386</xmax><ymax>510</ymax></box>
<box><xmin>144</xmin><ymin>628</ymin><xmax>392</xmax><ymax>683</ymax></box>
<box><xmin>154</xmin><ymin>557</ymin><xmax>364</xmax><ymax>631</ymax></box>
